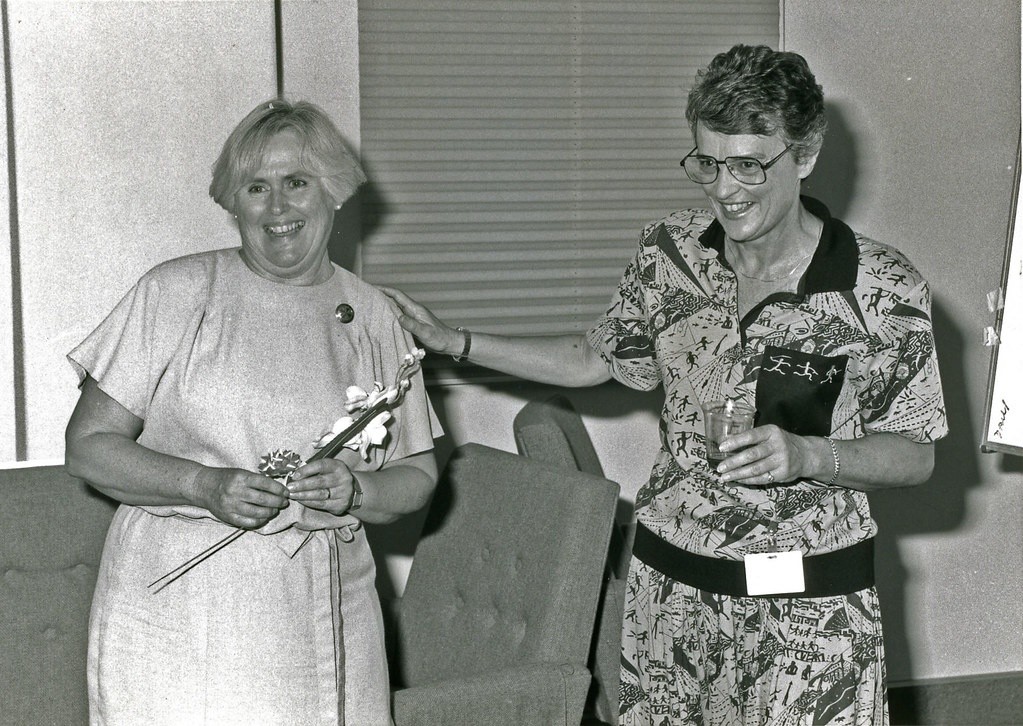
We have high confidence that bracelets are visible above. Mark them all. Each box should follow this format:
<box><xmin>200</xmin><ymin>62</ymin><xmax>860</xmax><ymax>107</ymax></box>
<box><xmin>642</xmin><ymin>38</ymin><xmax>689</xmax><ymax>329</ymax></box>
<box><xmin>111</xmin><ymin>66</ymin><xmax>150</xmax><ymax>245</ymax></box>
<box><xmin>823</xmin><ymin>435</ymin><xmax>840</xmax><ymax>485</ymax></box>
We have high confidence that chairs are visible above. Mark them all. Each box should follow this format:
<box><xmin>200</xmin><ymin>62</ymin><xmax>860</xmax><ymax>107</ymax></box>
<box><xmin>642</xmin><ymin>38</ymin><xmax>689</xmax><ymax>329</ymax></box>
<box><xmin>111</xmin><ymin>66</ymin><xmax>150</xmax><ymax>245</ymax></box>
<box><xmin>371</xmin><ymin>443</ymin><xmax>622</xmax><ymax>725</ymax></box>
<box><xmin>1</xmin><ymin>461</ymin><xmax>128</xmax><ymax>724</ymax></box>
<box><xmin>511</xmin><ymin>391</ymin><xmax>648</xmax><ymax>726</ymax></box>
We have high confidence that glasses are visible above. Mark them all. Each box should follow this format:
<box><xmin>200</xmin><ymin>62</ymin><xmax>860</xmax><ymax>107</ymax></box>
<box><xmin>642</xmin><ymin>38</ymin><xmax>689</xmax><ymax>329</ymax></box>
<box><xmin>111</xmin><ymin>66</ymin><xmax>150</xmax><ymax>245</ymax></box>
<box><xmin>680</xmin><ymin>141</ymin><xmax>797</xmax><ymax>186</ymax></box>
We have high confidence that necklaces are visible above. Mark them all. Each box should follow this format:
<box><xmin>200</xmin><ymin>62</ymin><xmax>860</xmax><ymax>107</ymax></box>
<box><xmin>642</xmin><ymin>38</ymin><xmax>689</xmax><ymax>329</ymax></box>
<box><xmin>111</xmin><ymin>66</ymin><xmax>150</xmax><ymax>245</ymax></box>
<box><xmin>725</xmin><ymin>232</ymin><xmax>822</xmax><ymax>283</ymax></box>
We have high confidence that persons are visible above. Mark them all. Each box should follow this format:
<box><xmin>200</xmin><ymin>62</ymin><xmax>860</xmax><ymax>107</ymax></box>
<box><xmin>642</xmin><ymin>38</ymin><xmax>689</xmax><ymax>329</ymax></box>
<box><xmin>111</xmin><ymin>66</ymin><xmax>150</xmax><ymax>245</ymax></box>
<box><xmin>373</xmin><ymin>43</ymin><xmax>951</xmax><ymax>726</ymax></box>
<box><xmin>65</xmin><ymin>97</ymin><xmax>445</xmax><ymax>726</ymax></box>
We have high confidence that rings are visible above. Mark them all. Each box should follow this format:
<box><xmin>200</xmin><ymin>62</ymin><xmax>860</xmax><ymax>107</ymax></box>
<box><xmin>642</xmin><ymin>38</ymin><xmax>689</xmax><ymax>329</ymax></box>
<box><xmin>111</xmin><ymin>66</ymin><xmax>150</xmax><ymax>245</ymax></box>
<box><xmin>325</xmin><ymin>488</ymin><xmax>331</xmax><ymax>501</ymax></box>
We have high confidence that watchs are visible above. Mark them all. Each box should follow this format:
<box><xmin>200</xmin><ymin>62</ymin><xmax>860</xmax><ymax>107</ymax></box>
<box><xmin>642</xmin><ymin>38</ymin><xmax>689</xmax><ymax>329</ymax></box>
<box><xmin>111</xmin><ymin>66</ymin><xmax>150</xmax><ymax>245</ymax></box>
<box><xmin>349</xmin><ymin>472</ymin><xmax>363</xmax><ymax>512</ymax></box>
<box><xmin>451</xmin><ymin>326</ymin><xmax>472</xmax><ymax>363</ymax></box>
